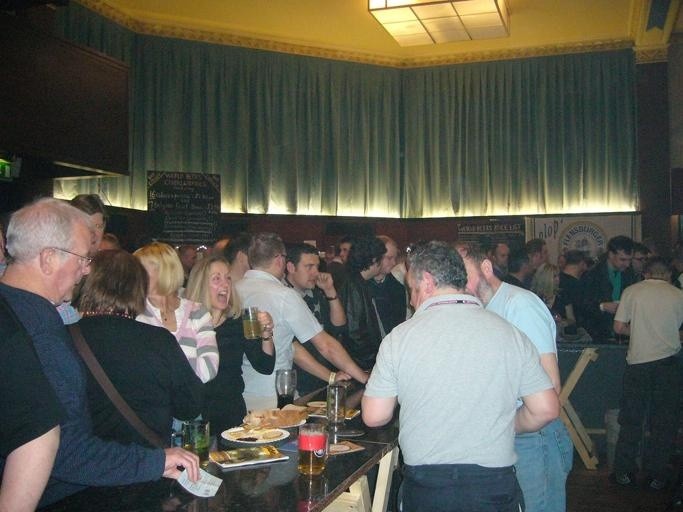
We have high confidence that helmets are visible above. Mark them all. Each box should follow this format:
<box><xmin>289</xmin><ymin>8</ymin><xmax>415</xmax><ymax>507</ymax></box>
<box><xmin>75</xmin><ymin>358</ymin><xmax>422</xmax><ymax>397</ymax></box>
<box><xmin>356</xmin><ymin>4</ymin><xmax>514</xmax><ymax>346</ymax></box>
<box><xmin>307</xmin><ymin>401</ymin><xmax>331</xmax><ymax>407</ymax></box>
<box><xmin>220</xmin><ymin>426</ymin><xmax>290</xmax><ymax>446</ymax></box>
<box><xmin>242</xmin><ymin>413</ymin><xmax>308</xmax><ymax>433</ymax></box>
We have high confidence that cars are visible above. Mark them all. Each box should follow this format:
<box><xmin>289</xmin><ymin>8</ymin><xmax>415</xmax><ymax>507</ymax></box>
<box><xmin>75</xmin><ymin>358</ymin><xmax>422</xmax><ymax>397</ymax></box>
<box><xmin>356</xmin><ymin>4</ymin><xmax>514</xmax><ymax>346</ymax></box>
<box><xmin>170</xmin><ymin>431</ymin><xmax>186</xmax><ymax>471</ymax></box>
<box><xmin>326</xmin><ymin>384</ymin><xmax>347</xmax><ymax>425</ymax></box>
<box><xmin>615</xmin><ymin>332</ymin><xmax>625</xmax><ymax>345</ymax></box>
<box><xmin>183</xmin><ymin>420</ymin><xmax>210</xmax><ymax>471</ymax></box>
<box><xmin>274</xmin><ymin>369</ymin><xmax>298</xmax><ymax>411</ymax></box>
<box><xmin>326</xmin><ymin>424</ymin><xmax>348</xmax><ymax>460</ymax></box>
<box><xmin>295</xmin><ymin>422</ymin><xmax>330</xmax><ymax>477</ymax></box>
<box><xmin>552</xmin><ymin>312</ymin><xmax>561</xmax><ymax>323</ymax></box>
<box><xmin>242</xmin><ymin>307</ymin><xmax>266</xmax><ymax>339</ymax></box>
<box><xmin>294</xmin><ymin>472</ymin><xmax>329</xmax><ymax>512</ymax></box>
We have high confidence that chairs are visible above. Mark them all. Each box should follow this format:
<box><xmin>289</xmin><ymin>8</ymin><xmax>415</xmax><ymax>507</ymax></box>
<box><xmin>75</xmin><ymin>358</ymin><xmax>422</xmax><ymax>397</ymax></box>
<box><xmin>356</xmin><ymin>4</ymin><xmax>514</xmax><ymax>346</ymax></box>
<box><xmin>607</xmin><ymin>470</ymin><xmax>675</xmax><ymax>493</ymax></box>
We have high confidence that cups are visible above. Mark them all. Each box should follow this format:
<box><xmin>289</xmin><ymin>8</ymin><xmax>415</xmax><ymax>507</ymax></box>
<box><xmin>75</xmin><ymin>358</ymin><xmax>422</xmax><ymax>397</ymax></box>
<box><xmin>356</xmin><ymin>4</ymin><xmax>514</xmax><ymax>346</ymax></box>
<box><xmin>38</xmin><ymin>245</ymin><xmax>97</xmax><ymax>269</ymax></box>
<box><xmin>274</xmin><ymin>252</ymin><xmax>291</xmax><ymax>261</ymax></box>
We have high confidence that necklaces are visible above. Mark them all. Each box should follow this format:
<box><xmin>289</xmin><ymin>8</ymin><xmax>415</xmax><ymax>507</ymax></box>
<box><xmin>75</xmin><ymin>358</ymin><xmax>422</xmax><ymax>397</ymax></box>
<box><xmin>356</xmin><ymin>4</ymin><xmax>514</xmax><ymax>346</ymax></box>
<box><xmin>158</xmin><ymin>299</ymin><xmax>181</xmax><ymax>321</ymax></box>
<box><xmin>377</xmin><ymin>276</ymin><xmax>385</xmax><ymax>283</ymax></box>
<box><xmin>80</xmin><ymin>311</ymin><xmax>135</xmax><ymax>320</ymax></box>
<box><xmin>423</xmin><ymin>299</ymin><xmax>480</xmax><ymax>311</ymax></box>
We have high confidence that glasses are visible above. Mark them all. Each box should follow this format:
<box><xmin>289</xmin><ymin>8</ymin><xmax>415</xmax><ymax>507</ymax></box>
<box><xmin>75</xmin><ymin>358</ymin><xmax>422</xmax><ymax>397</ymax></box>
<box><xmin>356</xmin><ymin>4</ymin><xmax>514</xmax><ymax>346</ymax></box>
<box><xmin>366</xmin><ymin>0</ymin><xmax>510</xmax><ymax>49</ymax></box>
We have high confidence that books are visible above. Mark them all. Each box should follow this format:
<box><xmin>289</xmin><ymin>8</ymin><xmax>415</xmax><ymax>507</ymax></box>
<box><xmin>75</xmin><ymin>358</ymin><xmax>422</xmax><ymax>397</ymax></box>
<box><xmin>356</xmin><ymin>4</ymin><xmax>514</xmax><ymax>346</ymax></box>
<box><xmin>208</xmin><ymin>445</ymin><xmax>290</xmax><ymax>469</ymax></box>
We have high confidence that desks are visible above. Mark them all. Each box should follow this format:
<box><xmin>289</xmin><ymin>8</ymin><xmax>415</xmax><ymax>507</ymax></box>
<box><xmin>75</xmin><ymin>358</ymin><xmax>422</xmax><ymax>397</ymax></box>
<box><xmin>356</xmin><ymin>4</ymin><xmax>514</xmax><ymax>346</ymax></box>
<box><xmin>555</xmin><ymin>342</ymin><xmax>629</xmax><ymax>471</ymax></box>
<box><xmin>93</xmin><ymin>428</ymin><xmax>394</xmax><ymax>512</ymax></box>
<box><xmin>294</xmin><ymin>365</ymin><xmax>402</xmax><ymax>467</ymax></box>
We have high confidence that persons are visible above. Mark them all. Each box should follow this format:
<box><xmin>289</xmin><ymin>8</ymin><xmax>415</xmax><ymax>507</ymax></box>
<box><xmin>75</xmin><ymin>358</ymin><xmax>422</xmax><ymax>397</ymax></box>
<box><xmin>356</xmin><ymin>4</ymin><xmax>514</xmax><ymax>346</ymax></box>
<box><xmin>184</xmin><ymin>230</ymin><xmax>414</xmax><ymax>449</ymax></box>
<box><xmin>1</xmin><ymin>193</ymin><xmax>219</xmax><ymax>512</ymax></box>
<box><xmin>233</xmin><ymin>456</ymin><xmax>299</xmax><ymax>509</ymax></box>
<box><xmin>361</xmin><ymin>234</ymin><xmax>681</xmax><ymax>510</ymax></box>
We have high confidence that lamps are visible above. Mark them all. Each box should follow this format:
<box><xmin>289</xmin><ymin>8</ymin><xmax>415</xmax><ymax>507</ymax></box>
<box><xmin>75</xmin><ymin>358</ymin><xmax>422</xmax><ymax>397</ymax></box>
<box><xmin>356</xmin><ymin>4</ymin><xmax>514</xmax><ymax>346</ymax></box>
<box><xmin>366</xmin><ymin>0</ymin><xmax>510</xmax><ymax>49</ymax></box>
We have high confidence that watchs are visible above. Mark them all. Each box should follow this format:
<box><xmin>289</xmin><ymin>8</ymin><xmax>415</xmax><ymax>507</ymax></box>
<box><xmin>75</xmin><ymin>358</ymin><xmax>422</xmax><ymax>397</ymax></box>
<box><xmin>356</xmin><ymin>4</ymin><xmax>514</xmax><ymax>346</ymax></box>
<box><xmin>263</xmin><ymin>331</ymin><xmax>273</xmax><ymax>341</ymax></box>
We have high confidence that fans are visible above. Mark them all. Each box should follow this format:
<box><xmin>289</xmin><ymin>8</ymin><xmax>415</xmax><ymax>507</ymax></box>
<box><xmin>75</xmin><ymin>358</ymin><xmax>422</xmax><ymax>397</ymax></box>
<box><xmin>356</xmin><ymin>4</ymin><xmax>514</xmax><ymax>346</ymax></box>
<box><xmin>147</xmin><ymin>170</ymin><xmax>221</xmax><ymax>251</ymax></box>
<box><xmin>457</xmin><ymin>222</ymin><xmax>524</xmax><ymax>248</ymax></box>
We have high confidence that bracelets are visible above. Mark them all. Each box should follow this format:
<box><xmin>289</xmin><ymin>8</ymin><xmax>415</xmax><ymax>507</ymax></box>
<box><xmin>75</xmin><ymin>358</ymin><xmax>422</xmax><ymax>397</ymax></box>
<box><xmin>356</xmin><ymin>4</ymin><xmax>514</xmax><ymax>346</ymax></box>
<box><xmin>329</xmin><ymin>372</ymin><xmax>336</xmax><ymax>384</ymax></box>
<box><xmin>600</xmin><ymin>303</ymin><xmax>606</xmax><ymax>311</ymax></box>
<box><xmin>327</xmin><ymin>293</ymin><xmax>338</xmax><ymax>300</ymax></box>
